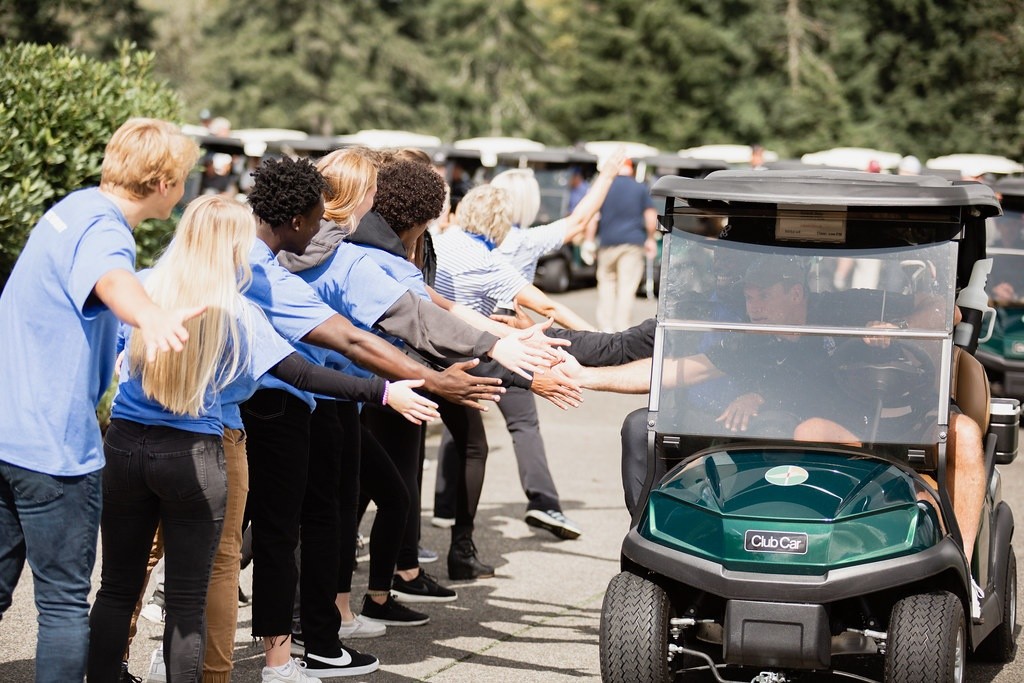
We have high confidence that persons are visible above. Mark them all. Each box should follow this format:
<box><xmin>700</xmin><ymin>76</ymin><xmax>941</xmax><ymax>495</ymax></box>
<box><xmin>132</xmin><ymin>140</ymin><xmax>627</xmax><ymax>682</ymax></box>
<box><xmin>86</xmin><ymin>194</ymin><xmax>439</xmax><ymax>683</ymax></box>
<box><xmin>486</xmin><ymin>227</ymin><xmax>987</xmax><ymax>621</ymax></box>
<box><xmin>983</xmin><ymin>210</ymin><xmax>1024</xmax><ymax>301</ymax></box>
<box><xmin>565</xmin><ymin>165</ymin><xmax>590</xmax><ymax>218</ymax></box>
<box><xmin>446</xmin><ymin>161</ymin><xmax>467</xmax><ymax>215</ymax></box>
<box><xmin>0</xmin><ymin>115</ymin><xmax>200</xmax><ymax>683</ymax></box>
<box><xmin>580</xmin><ymin>166</ymin><xmax>658</xmax><ymax>334</ymax></box>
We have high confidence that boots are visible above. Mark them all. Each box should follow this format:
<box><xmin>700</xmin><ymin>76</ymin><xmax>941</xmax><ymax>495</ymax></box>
<box><xmin>447</xmin><ymin>524</ymin><xmax>495</xmax><ymax>580</ymax></box>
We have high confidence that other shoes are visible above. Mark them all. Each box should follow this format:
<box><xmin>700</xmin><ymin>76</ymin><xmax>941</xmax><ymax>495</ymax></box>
<box><xmin>141</xmin><ymin>591</ymin><xmax>166</xmax><ymax>624</ymax></box>
<box><xmin>146</xmin><ymin>648</ymin><xmax>166</xmax><ymax>683</ymax></box>
<box><xmin>119</xmin><ymin>661</ymin><xmax>142</xmax><ymax>683</ymax></box>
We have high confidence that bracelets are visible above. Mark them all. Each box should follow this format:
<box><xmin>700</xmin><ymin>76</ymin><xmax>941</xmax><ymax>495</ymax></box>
<box><xmin>382</xmin><ymin>381</ymin><xmax>390</xmax><ymax>406</ymax></box>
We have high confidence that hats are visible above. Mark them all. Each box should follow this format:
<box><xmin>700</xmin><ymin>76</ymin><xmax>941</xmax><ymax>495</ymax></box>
<box><xmin>623</xmin><ymin>159</ymin><xmax>632</xmax><ymax>166</ymax></box>
<box><xmin>732</xmin><ymin>254</ymin><xmax>807</xmax><ymax>291</ymax></box>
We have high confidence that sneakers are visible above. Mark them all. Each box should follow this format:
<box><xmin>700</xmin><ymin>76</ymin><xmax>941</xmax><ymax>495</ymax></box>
<box><xmin>352</xmin><ymin>535</ymin><xmax>370</xmax><ymax>562</ymax></box>
<box><xmin>524</xmin><ymin>508</ymin><xmax>582</xmax><ymax>540</ymax></box>
<box><xmin>971</xmin><ymin>576</ymin><xmax>986</xmax><ymax>625</ymax></box>
<box><xmin>239</xmin><ymin>587</ymin><xmax>248</xmax><ymax>607</ymax></box>
<box><xmin>339</xmin><ymin>611</ymin><xmax>387</xmax><ymax>638</ymax></box>
<box><xmin>357</xmin><ymin>593</ymin><xmax>431</xmax><ymax>626</ymax></box>
<box><xmin>389</xmin><ymin>568</ymin><xmax>459</xmax><ymax>602</ymax></box>
<box><xmin>431</xmin><ymin>514</ymin><xmax>455</xmax><ymax>528</ymax></box>
<box><xmin>417</xmin><ymin>545</ymin><xmax>440</xmax><ymax>562</ymax></box>
<box><xmin>302</xmin><ymin>644</ymin><xmax>379</xmax><ymax>677</ymax></box>
<box><xmin>290</xmin><ymin>633</ymin><xmax>305</xmax><ymax>655</ymax></box>
<box><xmin>261</xmin><ymin>658</ymin><xmax>322</xmax><ymax>683</ymax></box>
